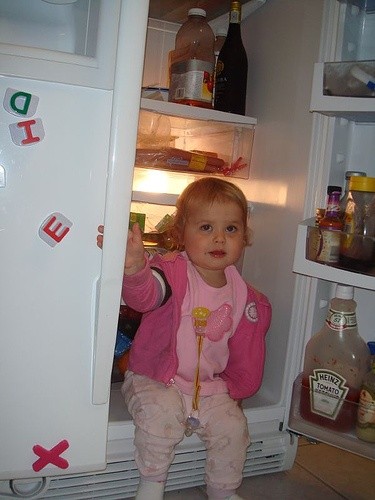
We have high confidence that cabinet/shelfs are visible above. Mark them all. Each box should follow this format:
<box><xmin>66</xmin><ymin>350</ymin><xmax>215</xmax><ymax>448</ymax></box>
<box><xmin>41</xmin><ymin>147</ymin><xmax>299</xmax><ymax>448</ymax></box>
<box><xmin>107</xmin><ymin>60</ymin><xmax>375</xmax><ymax>485</ymax></box>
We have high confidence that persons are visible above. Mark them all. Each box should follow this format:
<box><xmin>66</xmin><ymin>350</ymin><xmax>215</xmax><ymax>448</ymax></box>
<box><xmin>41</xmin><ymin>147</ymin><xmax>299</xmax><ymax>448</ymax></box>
<box><xmin>97</xmin><ymin>177</ymin><xmax>273</xmax><ymax>500</ymax></box>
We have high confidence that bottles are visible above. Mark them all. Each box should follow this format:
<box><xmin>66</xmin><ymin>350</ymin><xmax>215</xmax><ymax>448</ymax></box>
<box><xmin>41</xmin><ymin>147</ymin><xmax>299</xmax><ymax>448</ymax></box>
<box><xmin>319</xmin><ymin>186</ymin><xmax>343</xmax><ymax>265</ymax></box>
<box><xmin>214</xmin><ymin>3</ymin><xmax>249</xmax><ymax>114</ymax></box>
<box><xmin>298</xmin><ymin>284</ymin><xmax>371</xmax><ymax>429</ymax></box>
<box><xmin>213</xmin><ymin>24</ymin><xmax>227</xmax><ymax>66</ymax></box>
<box><xmin>168</xmin><ymin>4</ymin><xmax>212</xmax><ymax>105</ymax></box>
<box><xmin>337</xmin><ymin>171</ymin><xmax>366</xmax><ymax>210</ymax></box>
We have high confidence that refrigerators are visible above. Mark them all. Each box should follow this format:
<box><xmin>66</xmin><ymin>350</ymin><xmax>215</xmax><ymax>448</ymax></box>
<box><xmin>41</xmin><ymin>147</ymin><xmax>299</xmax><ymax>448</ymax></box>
<box><xmin>1</xmin><ymin>0</ymin><xmax>375</xmax><ymax>496</ymax></box>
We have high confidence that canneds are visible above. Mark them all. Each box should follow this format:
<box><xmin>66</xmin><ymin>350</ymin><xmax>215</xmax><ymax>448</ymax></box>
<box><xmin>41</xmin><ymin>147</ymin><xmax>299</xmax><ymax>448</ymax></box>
<box><xmin>338</xmin><ymin>176</ymin><xmax>375</xmax><ymax>275</ymax></box>
